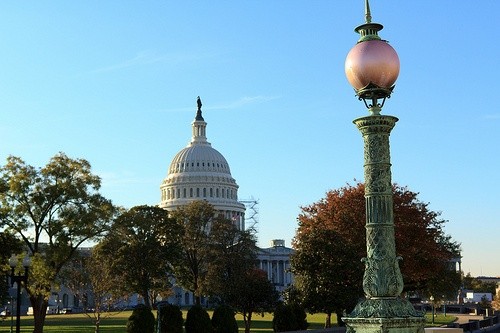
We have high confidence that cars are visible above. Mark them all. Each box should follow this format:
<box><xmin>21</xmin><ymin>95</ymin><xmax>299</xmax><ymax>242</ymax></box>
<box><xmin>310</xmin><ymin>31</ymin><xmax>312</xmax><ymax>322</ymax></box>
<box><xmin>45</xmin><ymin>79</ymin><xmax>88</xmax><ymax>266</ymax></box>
<box><xmin>26</xmin><ymin>304</ymin><xmax>128</xmax><ymax>316</ymax></box>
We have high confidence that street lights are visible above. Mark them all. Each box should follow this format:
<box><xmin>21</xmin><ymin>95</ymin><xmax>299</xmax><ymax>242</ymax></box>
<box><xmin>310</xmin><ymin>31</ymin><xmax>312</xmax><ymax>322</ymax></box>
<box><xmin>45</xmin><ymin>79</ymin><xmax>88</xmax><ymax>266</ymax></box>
<box><xmin>8</xmin><ymin>295</ymin><xmax>16</xmax><ymax>333</ymax></box>
<box><xmin>8</xmin><ymin>253</ymin><xmax>32</xmax><ymax>333</ymax></box>
<box><xmin>55</xmin><ymin>294</ymin><xmax>62</xmax><ymax>314</ymax></box>
